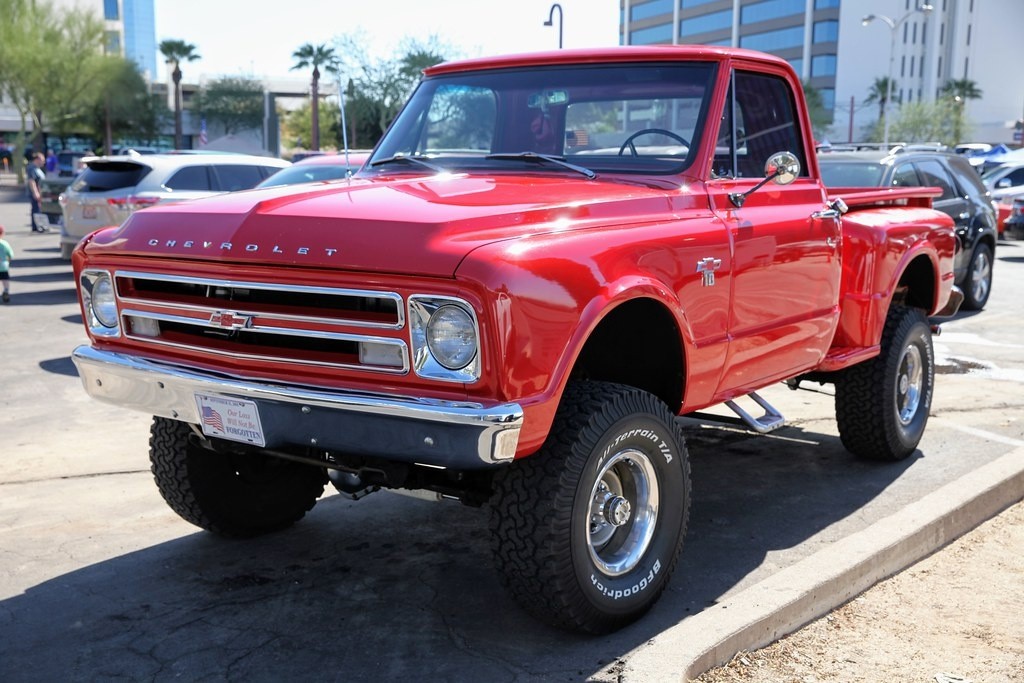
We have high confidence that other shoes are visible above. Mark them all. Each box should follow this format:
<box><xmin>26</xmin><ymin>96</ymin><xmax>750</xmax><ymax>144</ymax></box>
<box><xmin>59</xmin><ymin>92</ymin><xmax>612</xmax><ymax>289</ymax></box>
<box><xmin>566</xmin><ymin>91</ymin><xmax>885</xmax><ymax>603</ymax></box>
<box><xmin>3</xmin><ymin>292</ymin><xmax>10</xmax><ymax>302</ymax></box>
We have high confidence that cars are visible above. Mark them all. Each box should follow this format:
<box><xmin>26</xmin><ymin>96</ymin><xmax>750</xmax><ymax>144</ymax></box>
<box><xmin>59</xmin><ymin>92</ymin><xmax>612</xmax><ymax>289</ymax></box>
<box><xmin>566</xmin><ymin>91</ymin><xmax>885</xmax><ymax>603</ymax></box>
<box><xmin>574</xmin><ymin>137</ymin><xmax>1024</xmax><ymax>319</ymax></box>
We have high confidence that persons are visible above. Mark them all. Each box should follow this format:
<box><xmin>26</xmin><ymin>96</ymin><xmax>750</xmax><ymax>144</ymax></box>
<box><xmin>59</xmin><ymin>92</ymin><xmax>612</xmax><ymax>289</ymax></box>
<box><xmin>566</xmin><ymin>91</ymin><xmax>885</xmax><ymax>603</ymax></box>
<box><xmin>74</xmin><ymin>151</ymin><xmax>95</xmax><ymax>177</ymax></box>
<box><xmin>0</xmin><ymin>226</ymin><xmax>14</xmax><ymax>302</ymax></box>
<box><xmin>46</xmin><ymin>149</ymin><xmax>58</xmax><ymax>178</ymax></box>
<box><xmin>28</xmin><ymin>152</ymin><xmax>46</xmax><ymax>230</ymax></box>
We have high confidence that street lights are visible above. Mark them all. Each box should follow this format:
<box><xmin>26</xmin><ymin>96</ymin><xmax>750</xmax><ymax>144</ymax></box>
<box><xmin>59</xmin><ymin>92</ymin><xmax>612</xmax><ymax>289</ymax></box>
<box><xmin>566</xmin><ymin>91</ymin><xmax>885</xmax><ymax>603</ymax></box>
<box><xmin>543</xmin><ymin>2</ymin><xmax>564</xmax><ymax>50</ymax></box>
<box><xmin>860</xmin><ymin>3</ymin><xmax>936</xmax><ymax>156</ymax></box>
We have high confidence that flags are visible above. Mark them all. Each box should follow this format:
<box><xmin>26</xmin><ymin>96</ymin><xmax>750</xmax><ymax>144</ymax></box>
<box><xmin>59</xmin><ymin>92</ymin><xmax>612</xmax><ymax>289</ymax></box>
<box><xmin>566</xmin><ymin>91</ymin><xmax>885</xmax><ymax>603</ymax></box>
<box><xmin>198</xmin><ymin>116</ymin><xmax>208</xmax><ymax>147</ymax></box>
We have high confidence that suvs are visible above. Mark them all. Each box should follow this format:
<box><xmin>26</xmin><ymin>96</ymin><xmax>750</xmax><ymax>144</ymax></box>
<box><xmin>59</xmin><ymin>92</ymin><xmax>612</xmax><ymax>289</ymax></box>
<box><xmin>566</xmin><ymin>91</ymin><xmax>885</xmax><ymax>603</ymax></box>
<box><xmin>40</xmin><ymin>141</ymin><xmax>490</xmax><ymax>271</ymax></box>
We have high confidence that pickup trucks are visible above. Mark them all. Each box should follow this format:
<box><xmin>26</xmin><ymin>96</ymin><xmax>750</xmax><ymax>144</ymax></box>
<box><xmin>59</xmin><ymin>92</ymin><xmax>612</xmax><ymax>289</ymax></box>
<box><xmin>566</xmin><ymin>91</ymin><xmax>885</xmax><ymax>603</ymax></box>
<box><xmin>64</xmin><ymin>43</ymin><xmax>962</xmax><ymax>636</ymax></box>
